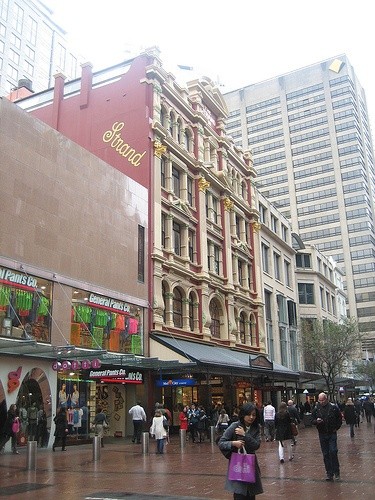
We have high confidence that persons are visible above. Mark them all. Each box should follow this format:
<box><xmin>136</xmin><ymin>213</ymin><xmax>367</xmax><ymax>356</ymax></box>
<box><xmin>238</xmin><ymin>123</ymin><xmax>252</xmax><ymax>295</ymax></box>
<box><xmin>52</xmin><ymin>407</ymin><xmax>69</xmax><ymax>452</ymax></box>
<box><xmin>300</xmin><ymin>399</ymin><xmax>318</xmax><ymax>413</ymax></box>
<box><xmin>310</xmin><ymin>392</ymin><xmax>342</xmax><ymax>480</ymax></box>
<box><xmin>343</xmin><ymin>397</ymin><xmax>375</xmax><ymax>436</ymax></box>
<box><xmin>179</xmin><ymin>399</ymin><xmax>240</xmax><ymax>454</ymax></box>
<box><xmin>286</xmin><ymin>400</ymin><xmax>301</xmax><ymax>446</ymax></box>
<box><xmin>275</xmin><ymin>402</ymin><xmax>293</xmax><ymax>463</ymax></box>
<box><xmin>263</xmin><ymin>401</ymin><xmax>276</xmax><ymax>442</ymax></box>
<box><xmin>91</xmin><ymin>407</ymin><xmax>109</xmax><ymax>448</ymax></box>
<box><xmin>218</xmin><ymin>402</ymin><xmax>264</xmax><ymax>500</ymax></box>
<box><xmin>150</xmin><ymin>401</ymin><xmax>172</xmax><ymax>454</ymax></box>
<box><xmin>0</xmin><ymin>404</ymin><xmax>21</xmax><ymax>455</ymax></box>
<box><xmin>129</xmin><ymin>400</ymin><xmax>147</xmax><ymax>444</ymax></box>
<box><xmin>329</xmin><ymin>399</ymin><xmax>347</xmax><ymax>412</ymax></box>
<box><xmin>59</xmin><ymin>383</ymin><xmax>80</xmax><ymax>410</ymax></box>
<box><xmin>19</xmin><ymin>400</ymin><xmax>45</xmax><ymax>447</ymax></box>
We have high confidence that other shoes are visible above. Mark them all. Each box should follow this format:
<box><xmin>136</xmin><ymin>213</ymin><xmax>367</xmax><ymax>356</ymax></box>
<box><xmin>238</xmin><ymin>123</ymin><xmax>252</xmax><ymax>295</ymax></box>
<box><xmin>53</xmin><ymin>446</ymin><xmax>55</xmax><ymax>451</ymax></box>
<box><xmin>280</xmin><ymin>459</ymin><xmax>284</xmax><ymax>463</ymax></box>
<box><xmin>62</xmin><ymin>449</ymin><xmax>67</xmax><ymax>451</ymax></box>
<box><xmin>266</xmin><ymin>438</ymin><xmax>269</xmax><ymax>442</ymax></box>
<box><xmin>289</xmin><ymin>455</ymin><xmax>295</xmax><ymax>461</ymax></box>
<box><xmin>336</xmin><ymin>474</ymin><xmax>340</xmax><ymax>479</ymax></box>
<box><xmin>137</xmin><ymin>442</ymin><xmax>142</xmax><ymax>444</ymax></box>
<box><xmin>326</xmin><ymin>477</ymin><xmax>333</xmax><ymax>480</ymax></box>
<box><xmin>14</xmin><ymin>451</ymin><xmax>20</xmax><ymax>454</ymax></box>
<box><xmin>294</xmin><ymin>440</ymin><xmax>297</xmax><ymax>445</ymax></box>
<box><xmin>132</xmin><ymin>440</ymin><xmax>135</xmax><ymax>443</ymax></box>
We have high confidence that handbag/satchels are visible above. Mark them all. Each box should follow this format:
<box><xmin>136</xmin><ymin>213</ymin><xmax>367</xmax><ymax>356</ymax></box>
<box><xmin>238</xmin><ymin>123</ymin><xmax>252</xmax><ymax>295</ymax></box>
<box><xmin>228</xmin><ymin>445</ymin><xmax>256</xmax><ymax>483</ymax></box>
<box><xmin>216</xmin><ymin>422</ymin><xmax>242</xmax><ymax>460</ymax></box>
<box><xmin>12</xmin><ymin>417</ymin><xmax>20</xmax><ymax>432</ymax></box>
<box><xmin>163</xmin><ymin>416</ymin><xmax>168</xmax><ymax>429</ymax></box>
<box><xmin>291</xmin><ymin>422</ymin><xmax>298</xmax><ymax>436</ymax></box>
<box><xmin>103</xmin><ymin>420</ymin><xmax>110</xmax><ymax>429</ymax></box>
<box><xmin>54</xmin><ymin>416</ymin><xmax>58</xmax><ymax>422</ymax></box>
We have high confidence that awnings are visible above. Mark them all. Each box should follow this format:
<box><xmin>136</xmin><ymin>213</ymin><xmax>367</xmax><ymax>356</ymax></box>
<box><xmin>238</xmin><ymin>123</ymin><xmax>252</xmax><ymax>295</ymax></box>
<box><xmin>297</xmin><ymin>369</ymin><xmax>368</xmax><ymax>386</ymax></box>
<box><xmin>150</xmin><ymin>333</ymin><xmax>298</xmax><ymax>375</ymax></box>
